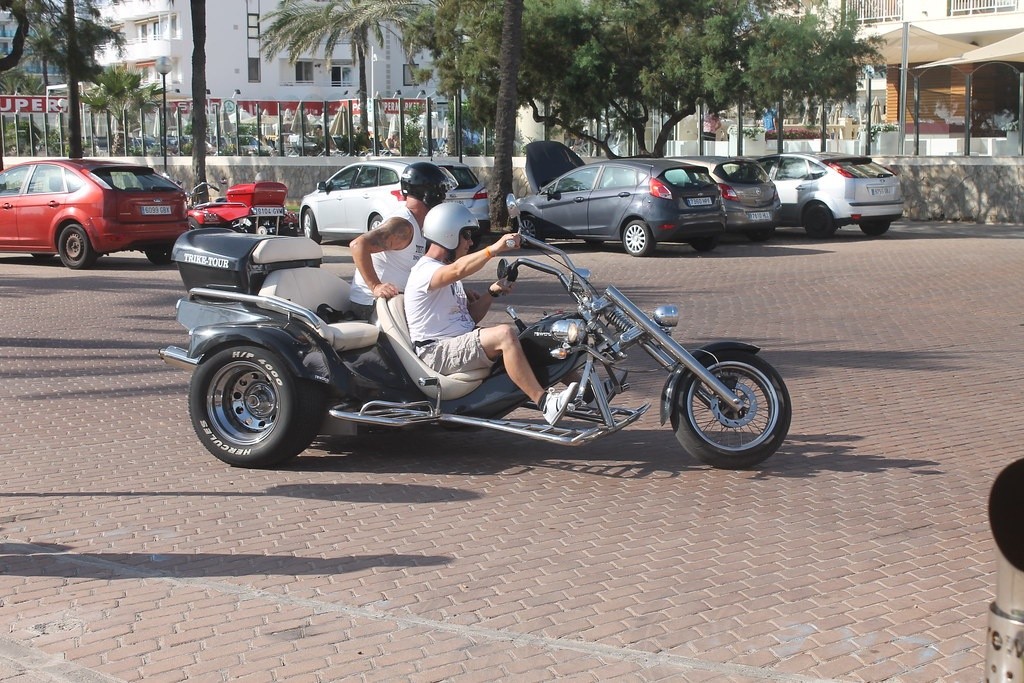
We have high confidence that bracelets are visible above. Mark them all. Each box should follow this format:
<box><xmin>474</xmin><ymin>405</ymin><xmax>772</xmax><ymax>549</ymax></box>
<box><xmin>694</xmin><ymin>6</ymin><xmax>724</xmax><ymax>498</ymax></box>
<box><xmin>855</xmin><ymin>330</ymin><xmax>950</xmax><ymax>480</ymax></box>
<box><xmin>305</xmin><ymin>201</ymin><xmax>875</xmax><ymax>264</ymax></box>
<box><xmin>485</xmin><ymin>245</ymin><xmax>495</xmax><ymax>258</ymax></box>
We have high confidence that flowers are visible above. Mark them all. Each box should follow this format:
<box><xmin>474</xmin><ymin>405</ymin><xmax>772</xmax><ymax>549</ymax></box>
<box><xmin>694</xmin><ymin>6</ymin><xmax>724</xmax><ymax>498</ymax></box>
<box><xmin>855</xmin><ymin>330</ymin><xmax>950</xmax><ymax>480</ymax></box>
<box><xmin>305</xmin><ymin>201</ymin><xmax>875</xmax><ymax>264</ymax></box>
<box><xmin>859</xmin><ymin>123</ymin><xmax>898</xmax><ymax>142</ymax></box>
<box><xmin>729</xmin><ymin>125</ymin><xmax>766</xmax><ymax>141</ymax></box>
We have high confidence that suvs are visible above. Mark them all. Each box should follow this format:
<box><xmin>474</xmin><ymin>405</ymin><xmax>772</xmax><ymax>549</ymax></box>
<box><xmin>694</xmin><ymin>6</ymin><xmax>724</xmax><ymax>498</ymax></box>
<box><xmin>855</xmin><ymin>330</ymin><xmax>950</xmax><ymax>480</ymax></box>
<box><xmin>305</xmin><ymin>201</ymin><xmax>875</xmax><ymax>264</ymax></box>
<box><xmin>756</xmin><ymin>151</ymin><xmax>906</xmax><ymax>239</ymax></box>
<box><xmin>298</xmin><ymin>159</ymin><xmax>491</xmax><ymax>244</ymax></box>
<box><xmin>507</xmin><ymin>140</ymin><xmax>728</xmax><ymax>257</ymax></box>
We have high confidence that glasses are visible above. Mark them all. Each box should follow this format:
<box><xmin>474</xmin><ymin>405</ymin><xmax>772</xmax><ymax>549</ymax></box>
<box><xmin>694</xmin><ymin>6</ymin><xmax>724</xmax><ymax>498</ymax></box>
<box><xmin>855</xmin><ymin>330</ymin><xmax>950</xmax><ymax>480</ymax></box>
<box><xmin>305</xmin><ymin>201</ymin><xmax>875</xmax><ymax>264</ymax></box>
<box><xmin>461</xmin><ymin>231</ymin><xmax>473</xmax><ymax>240</ymax></box>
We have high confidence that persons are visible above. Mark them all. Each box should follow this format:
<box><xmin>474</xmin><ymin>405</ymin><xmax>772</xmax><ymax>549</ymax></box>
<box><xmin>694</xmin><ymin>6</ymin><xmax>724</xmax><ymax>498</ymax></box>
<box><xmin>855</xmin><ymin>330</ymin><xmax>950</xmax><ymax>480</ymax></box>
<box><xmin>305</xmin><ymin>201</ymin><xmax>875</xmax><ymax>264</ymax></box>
<box><xmin>349</xmin><ymin>162</ymin><xmax>482</xmax><ymax>321</ymax></box>
<box><xmin>703</xmin><ymin>96</ymin><xmax>721</xmax><ymax>141</ymax></box>
<box><xmin>313</xmin><ymin>125</ymin><xmax>324</xmax><ymax>152</ymax></box>
<box><xmin>404</xmin><ymin>201</ymin><xmax>628</xmax><ymax>428</ymax></box>
<box><xmin>761</xmin><ymin>104</ymin><xmax>780</xmax><ymax>133</ymax></box>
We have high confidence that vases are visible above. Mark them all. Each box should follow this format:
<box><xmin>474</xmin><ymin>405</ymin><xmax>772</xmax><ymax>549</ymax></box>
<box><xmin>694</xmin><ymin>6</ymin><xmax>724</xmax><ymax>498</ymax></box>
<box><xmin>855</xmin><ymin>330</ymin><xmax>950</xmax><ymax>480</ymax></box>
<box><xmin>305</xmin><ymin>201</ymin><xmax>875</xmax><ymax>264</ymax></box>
<box><xmin>730</xmin><ymin>134</ymin><xmax>765</xmax><ymax>156</ymax></box>
<box><xmin>859</xmin><ymin>131</ymin><xmax>899</xmax><ymax>155</ymax></box>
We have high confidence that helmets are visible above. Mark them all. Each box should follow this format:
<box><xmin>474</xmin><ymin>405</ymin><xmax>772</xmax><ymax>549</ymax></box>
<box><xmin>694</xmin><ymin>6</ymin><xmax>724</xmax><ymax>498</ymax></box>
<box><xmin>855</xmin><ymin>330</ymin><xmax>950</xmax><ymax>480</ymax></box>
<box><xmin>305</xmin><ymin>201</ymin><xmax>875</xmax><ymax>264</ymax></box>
<box><xmin>421</xmin><ymin>202</ymin><xmax>480</xmax><ymax>251</ymax></box>
<box><xmin>401</xmin><ymin>162</ymin><xmax>445</xmax><ymax>208</ymax></box>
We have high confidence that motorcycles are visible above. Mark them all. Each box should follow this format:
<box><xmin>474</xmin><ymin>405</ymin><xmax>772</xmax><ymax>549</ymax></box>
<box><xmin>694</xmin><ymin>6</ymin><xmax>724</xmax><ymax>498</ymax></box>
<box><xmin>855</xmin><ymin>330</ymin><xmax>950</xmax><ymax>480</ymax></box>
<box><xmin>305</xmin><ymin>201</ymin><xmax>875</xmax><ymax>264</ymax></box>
<box><xmin>162</xmin><ymin>172</ymin><xmax>300</xmax><ymax>235</ymax></box>
<box><xmin>158</xmin><ymin>226</ymin><xmax>793</xmax><ymax>469</ymax></box>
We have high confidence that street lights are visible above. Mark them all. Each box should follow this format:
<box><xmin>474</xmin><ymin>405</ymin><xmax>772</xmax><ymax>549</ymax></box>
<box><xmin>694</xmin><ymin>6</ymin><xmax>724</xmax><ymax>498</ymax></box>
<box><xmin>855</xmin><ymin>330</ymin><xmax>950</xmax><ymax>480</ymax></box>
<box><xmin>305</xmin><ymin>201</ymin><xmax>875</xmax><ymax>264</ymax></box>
<box><xmin>155</xmin><ymin>56</ymin><xmax>174</xmax><ymax>174</ymax></box>
<box><xmin>371</xmin><ymin>45</ymin><xmax>379</xmax><ymax>97</ymax></box>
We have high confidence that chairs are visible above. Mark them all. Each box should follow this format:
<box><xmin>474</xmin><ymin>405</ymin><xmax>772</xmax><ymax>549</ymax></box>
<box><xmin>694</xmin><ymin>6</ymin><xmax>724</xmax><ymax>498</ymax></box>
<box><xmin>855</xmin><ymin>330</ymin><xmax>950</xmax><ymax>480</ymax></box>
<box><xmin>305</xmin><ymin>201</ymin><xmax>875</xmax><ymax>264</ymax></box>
<box><xmin>125</xmin><ymin>132</ymin><xmax>448</xmax><ymax>157</ymax></box>
<box><xmin>375</xmin><ymin>295</ymin><xmax>491</xmax><ymax>401</ymax></box>
<box><xmin>249</xmin><ymin>236</ymin><xmax>381</xmax><ymax>354</ymax></box>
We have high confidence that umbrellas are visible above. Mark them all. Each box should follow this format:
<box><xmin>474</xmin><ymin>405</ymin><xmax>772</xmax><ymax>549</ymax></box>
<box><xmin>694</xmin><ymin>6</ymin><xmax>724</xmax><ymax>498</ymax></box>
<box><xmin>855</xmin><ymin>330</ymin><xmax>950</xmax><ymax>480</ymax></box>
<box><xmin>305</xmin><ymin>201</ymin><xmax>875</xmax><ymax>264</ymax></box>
<box><xmin>801</xmin><ymin>96</ymin><xmax>882</xmax><ymax>125</ymax></box>
<box><xmin>290</xmin><ymin>100</ymin><xmax>311</xmax><ymax>150</ymax></box>
<box><xmin>153</xmin><ymin>107</ymin><xmax>166</xmax><ymax>150</ymax></box>
<box><xmin>220</xmin><ymin>102</ymin><xmax>233</xmax><ymax>145</ymax></box>
<box><xmin>330</xmin><ymin>104</ymin><xmax>348</xmax><ymax>142</ymax></box>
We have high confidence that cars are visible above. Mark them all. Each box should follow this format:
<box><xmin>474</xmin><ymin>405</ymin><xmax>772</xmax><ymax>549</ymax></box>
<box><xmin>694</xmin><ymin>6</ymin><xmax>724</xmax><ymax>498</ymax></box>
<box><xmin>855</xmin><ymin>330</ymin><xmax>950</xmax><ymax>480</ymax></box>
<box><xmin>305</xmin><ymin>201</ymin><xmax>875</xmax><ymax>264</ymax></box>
<box><xmin>238</xmin><ymin>134</ymin><xmax>275</xmax><ymax>155</ymax></box>
<box><xmin>665</xmin><ymin>156</ymin><xmax>783</xmax><ymax>241</ymax></box>
<box><xmin>124</xmin><ymin>135</ymin><xmax>237</xmax><ymax>155</ymax></box>
<box><xmin>0</xmin><ymin>159</ymin><xmax>189</xmax><ymax>270</ymax></box>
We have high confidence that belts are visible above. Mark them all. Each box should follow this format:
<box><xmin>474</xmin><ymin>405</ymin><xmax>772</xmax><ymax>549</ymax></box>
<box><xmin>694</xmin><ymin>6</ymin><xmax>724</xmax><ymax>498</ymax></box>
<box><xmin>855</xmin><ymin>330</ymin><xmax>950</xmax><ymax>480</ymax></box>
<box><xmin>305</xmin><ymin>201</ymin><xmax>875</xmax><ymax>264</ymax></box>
<box><xmin>415</xmin><ymin>339</ymin><xmax>435</xmax><ymax>348</ymax></box>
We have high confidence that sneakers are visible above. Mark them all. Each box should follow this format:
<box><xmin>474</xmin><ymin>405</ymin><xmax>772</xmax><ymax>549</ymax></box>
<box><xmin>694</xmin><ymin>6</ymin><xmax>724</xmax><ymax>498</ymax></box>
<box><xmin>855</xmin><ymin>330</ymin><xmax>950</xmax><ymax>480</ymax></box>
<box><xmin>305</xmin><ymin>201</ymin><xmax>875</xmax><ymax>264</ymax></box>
<box><xmin>586</xmin><ymin>368</ymin><xmax>628</xmax><ymax>415</ymax></box>
<box><xmin>543</xmin><ymin>382</ymin><xmax>579</xmax><ymax>426</ymax></box>
